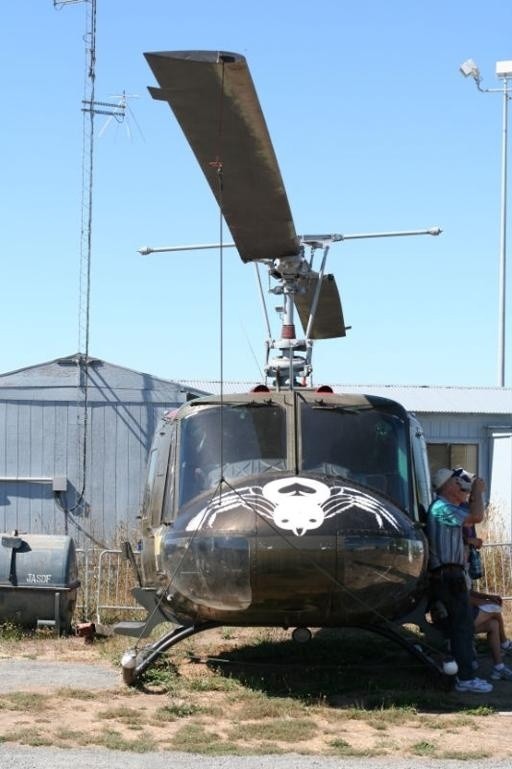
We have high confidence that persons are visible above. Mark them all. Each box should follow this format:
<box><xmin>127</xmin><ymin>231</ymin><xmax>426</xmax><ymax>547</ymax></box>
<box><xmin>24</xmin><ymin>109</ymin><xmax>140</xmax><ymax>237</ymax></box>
<box><xmin>426</xmin><ymin>469</ymin><xmax>512</xmax><ymax>694</ymax></box>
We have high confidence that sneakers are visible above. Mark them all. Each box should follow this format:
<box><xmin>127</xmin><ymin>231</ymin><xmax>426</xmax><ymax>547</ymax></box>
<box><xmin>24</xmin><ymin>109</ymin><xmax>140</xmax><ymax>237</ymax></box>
<box><xmin>500</xmin><ymin>638</ymin><xmax>512</xmax><ymax>656</ymax></box>
<box><xmin>455</xmin><ymin>675</ymin><xmax>494</xmax><ymax>693</ymax></box>
<box><xmin>490</xmin><ymin>665</ymin><xmax>512</xmax><ymax>680</ymax></box>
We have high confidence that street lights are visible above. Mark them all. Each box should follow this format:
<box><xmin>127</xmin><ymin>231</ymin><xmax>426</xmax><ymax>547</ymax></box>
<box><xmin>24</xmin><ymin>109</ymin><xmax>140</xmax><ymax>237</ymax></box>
<box><xmin>458</xmin><ymin>58</ymin><xmax>511</xmax><ymax>389</ymax></box>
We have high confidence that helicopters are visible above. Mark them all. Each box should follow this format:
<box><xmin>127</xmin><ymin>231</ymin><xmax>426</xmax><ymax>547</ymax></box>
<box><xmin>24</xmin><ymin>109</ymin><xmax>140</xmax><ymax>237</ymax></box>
<box><xmin>113</xmin><ymin>44</ymin><xmax>473</xmax><ymax>687</ymax></box>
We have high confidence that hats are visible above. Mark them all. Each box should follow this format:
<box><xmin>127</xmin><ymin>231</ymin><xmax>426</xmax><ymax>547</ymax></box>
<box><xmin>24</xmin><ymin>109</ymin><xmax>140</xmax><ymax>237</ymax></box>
<box><xmin>431</xmin><ymin>468</ymin><xmax>462</xmax><ymax>491</ymax></box>
